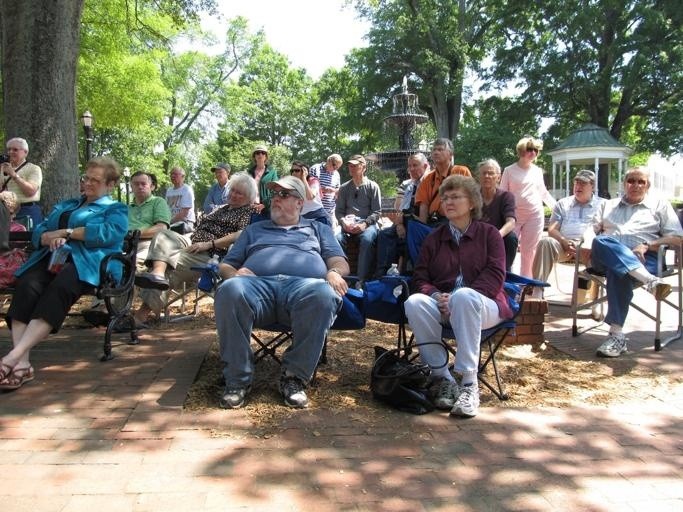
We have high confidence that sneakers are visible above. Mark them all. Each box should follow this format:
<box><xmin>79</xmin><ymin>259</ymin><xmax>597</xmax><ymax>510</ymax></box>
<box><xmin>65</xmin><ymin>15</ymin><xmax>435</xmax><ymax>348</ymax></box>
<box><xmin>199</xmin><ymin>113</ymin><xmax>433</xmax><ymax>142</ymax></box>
<box><xmin>450</xmin><ymin>383</ymin><xmax>481</xmax><ymax>418</ymax></box>
<box><xmin>220</xmin><ymin>369</ymin><xmax>254</xmax><ymax>409</ymax></box>
<box><xmin>278</xmin><ymin>365</ymin><xmax>309</xmax><ymax>408</ymax></box>
<box><xmin>596</xmin><ymin>333</ymin><xmax>628</xmax><ymax>358</ymax></box>
<box><xmin>134</xmin><ymin>271</ymin><xmax>170</xmax><ymax>292</ymax></box>
<box><xmin>434</xmin><ymin>377</ymin><xmax>459</xmax><ymax>410</ymax></box>
<box><xmin>643</xmin><ymin>274</ymin><xmax>673</xmax><ymax>301</ymax></box>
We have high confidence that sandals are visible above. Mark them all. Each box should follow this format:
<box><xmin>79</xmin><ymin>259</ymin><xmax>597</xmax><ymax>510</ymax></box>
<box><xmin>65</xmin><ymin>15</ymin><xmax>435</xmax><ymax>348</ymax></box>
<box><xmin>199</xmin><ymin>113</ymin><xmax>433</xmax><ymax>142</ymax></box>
<box><xmin>1</xmin><ymin>364</ymin><xmax>35</xmax><ymax>390</ymax></box>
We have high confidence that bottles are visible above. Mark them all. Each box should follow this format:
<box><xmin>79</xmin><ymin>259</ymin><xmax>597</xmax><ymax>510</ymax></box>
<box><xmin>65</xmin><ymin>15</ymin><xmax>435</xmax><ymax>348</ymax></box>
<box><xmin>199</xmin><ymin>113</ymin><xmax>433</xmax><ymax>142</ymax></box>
<box><xmin>387</xmin><ymin>263</ymin><xmax>400</xmax><ymax>276</ymax></box>
<box><xmin>199</xmin><ymin>254</ymin><xmax>220</xmax><ymax>295</ymax></box>
<box><xmin>48</xmin><ymin>244</ymin><xmax>73</xmax><ymax>273</ymax></box>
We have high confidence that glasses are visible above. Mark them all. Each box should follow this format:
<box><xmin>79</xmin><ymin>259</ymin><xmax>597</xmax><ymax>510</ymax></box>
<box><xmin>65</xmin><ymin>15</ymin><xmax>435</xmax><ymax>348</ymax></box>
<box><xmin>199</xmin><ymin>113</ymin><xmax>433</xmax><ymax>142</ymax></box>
<box><xmin>526</xmin><ymin>147</ymin><xmax>539</xmax><ymax>153</ymax></box>
<box><xmin>255</xmin><ymin>151</ymin><xmax>266</xmax><ymax>155</ymax></box>
<box><xmin>626</xmin><ymin>178</ymin><xmax>647</xmax><ymax>185</ymax></box>
<box><xmin>290</xmin><ymin>168</ymin><xmax>301</xmax><ymax>173</ymax></box>
<box><xmin>81</xmin><ymin>175</ymin><xmax>100</xmax><ymax>186</ymax></box>
<box><xmin>270</xmin><ymin>190</ymin><xmax>290</xmax><ymax>198</ymax></box>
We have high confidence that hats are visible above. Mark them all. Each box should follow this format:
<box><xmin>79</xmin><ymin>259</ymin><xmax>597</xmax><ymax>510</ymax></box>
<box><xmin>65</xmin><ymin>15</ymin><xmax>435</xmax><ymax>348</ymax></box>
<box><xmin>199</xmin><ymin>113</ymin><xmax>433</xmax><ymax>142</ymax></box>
<box><xmin>345</xmin><ymin>154</ymin><xmax>366</xmax><ymax>165</ymax></box>
<box><xmin>252</xmin><ymin>145</ymin><xmax>268</xmax><ymax>153</ymax></box>
<box><xmin>574</xmin><ymin>169</ymin><xmax>597</xmax><ymax>183</ymax></box>
<box><xmin>211</xmin><ymin>162</ymin><xmax>231</xmax><ymax>173</ymax></box>
<box><xmin>266</xmin><ymin>175</ymin><xmax>306</xmax><ymax>201</ymax></box>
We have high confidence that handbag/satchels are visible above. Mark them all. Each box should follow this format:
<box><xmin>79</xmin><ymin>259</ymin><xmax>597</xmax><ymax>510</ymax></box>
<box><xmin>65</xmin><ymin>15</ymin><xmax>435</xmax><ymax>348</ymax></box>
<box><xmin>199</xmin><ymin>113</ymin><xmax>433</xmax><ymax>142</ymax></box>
<box><xmin>370</xmin><ymin>339</ymin><xmax>450</xmax><ymax>416</ymax></box>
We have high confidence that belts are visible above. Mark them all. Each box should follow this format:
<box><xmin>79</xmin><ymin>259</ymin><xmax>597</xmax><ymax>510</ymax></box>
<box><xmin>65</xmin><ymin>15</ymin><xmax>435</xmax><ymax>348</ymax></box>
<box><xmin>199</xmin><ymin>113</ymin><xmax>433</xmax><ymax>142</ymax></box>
<box><xmin>20</xmin><ymin>201</ymin><xmax>40</xmax><ymax>207</ymax></box>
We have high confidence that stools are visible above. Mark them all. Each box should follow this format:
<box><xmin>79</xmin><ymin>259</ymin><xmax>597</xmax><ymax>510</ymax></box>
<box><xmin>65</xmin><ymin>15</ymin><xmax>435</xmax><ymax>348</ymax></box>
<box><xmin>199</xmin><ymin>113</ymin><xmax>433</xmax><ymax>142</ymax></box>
<box><xmin>544</xmin><ymin>256</ymin><xmax>607</xmax><ymax>321</ymax></box>
<box><xmin>155</xmin><ymin>273</ymin><xmax>215</xmax><ymax>324</ymax></box>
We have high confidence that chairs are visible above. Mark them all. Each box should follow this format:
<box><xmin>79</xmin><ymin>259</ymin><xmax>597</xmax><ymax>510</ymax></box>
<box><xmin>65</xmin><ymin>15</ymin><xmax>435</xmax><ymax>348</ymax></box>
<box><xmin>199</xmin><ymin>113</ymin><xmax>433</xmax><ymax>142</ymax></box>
<box><xmin>360</xmin><ymin>216</ymin><xmax>550</xmax><ymax>401</ymax></box>
<box><xmin>189</xmin><ymin>210</ymin><xmax>365</xmax><ymax>388</ymax></box>
<box><xmin>569</xmin><ymin>203</ymin><xmax>682</xmax><ymax>353</ymax></box>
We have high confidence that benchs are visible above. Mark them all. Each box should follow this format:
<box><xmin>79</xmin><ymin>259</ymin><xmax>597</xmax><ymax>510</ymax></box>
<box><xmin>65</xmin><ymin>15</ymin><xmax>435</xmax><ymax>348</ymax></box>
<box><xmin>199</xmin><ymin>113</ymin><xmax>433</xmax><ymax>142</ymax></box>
<box><xmin>0</xmin><ymin>227</ymin><xmax>146</xmax><ymax>363</ymax></box>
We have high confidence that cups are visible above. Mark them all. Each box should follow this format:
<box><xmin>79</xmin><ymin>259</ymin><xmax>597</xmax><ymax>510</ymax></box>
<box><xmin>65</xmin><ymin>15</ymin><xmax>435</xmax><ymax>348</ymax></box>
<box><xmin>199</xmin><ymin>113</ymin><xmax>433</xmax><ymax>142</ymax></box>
<box><xmin>346</xmin><ymin>215</ymin><xmax>357</xmax><ymax>229</ymax></box>
